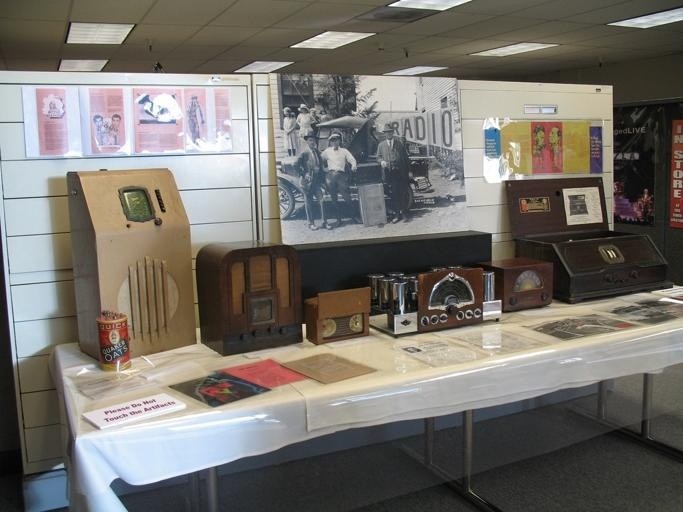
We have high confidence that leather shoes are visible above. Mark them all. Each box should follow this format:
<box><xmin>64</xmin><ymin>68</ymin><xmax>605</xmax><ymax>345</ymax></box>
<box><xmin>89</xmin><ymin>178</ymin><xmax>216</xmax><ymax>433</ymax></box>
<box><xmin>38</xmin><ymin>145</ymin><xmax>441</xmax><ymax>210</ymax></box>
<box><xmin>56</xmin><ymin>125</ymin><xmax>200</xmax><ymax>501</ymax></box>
<box><xmin>309</xmin><ymin>216</ymin><xmax>362</xmax><ymax>230</ymax></box>
<box><xmin>392</xmin><ymin>212</ymin><xmax>408</xmax><ymax>223</ymax></box>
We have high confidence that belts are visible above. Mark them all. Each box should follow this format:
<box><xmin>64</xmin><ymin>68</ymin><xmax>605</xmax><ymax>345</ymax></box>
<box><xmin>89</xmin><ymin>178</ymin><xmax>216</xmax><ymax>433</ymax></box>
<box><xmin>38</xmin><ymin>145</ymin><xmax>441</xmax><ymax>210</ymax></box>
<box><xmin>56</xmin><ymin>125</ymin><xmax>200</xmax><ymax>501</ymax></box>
<box><xmin>329</xmin><ymin>170</ymin><xmax>345</xmax><ymax>174</ymax></box>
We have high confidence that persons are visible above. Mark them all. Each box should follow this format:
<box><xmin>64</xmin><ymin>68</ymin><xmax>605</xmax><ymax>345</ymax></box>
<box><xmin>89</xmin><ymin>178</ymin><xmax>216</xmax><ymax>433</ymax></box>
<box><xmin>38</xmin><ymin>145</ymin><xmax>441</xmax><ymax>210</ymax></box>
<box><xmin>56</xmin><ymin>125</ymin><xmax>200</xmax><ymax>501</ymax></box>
<box><xmin>308</xmin><ymin>107</ymin><xmax>321</xmax><ymax>141</ymax></box>
<box><xmin>184</xmin><ymin>94</ymin><xmax>205</xmax><ymax>145</ymax></box>
<box><xmin>281</xmin><ymin>106</ymin><xmax>299</xmax><ymax>157</ymax></box>
<box><xmin>93</xmin><ymin>115</ymin><xmax>106</xmax><ymax>146</ymax></box>
<box><xmin>294</xmin><ymin>103</ymin><xmax>318</xmax><ymax>145</ymax></box>
<box><xmin>108</xmin><ymin>113</ymin><xmax>123</xmax><ymax>146</ymax></box>
<box><xmin>319</xmin><ymin>133</ymin><xmax>363</xmax><ymax>227</ymax></box>
<box><xmin>291</xmin><ymin>130</ymin><xmax>333</xmax><ymax>232</ymax></box>
<box><xmin>374</xmin><ymin>123</ymin><xmax>412</xmax><ymax>224</ymax></box>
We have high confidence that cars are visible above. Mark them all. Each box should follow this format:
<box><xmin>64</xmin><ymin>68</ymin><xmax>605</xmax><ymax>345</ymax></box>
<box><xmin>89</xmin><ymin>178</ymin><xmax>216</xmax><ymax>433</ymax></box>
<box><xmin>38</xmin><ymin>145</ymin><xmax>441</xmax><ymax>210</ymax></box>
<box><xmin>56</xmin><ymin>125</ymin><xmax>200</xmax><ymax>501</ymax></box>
<box><xmin>272</xmin><ymin>116</ymin><xmax>418</xmax><ymax>219</ymax></box>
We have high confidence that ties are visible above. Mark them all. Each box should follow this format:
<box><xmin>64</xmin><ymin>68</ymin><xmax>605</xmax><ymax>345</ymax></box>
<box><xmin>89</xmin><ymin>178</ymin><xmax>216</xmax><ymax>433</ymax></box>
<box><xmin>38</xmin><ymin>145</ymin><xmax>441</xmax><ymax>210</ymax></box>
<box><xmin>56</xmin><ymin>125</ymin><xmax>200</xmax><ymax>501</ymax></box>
<box><xmin>313</xmin><ymin>150</ymin><xmax>317</xmax><ymax>166</ymax></box>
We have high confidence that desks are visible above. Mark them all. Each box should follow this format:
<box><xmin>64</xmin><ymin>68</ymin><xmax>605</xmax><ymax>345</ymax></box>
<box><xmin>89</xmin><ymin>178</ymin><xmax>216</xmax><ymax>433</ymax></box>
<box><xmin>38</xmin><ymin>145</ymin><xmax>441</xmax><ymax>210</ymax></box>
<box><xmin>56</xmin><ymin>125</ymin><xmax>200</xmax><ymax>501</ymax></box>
<box><xmin>51</xmin><ymin>284</ymin><xmax>681</xmax><ymax>511</ymax></box>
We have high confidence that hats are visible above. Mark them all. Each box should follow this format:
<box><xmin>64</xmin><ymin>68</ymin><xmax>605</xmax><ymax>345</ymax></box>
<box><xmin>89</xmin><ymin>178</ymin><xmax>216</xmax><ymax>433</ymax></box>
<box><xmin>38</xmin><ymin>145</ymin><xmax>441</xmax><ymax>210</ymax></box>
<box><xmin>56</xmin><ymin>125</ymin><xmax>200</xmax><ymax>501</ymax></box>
<box><xmin>328</xmin><ymin>134</ymin><xmax>340</xmax><ymax>140</ymax></box>
<box><xmin>303</xmin><ymin>132</ymin><xmax>318</xmax><ymax>141</ymax></box>
<box><xmin>298</xmin><ymin>104</ymin><xmax>308</xmax><ymax>112</ymax></box>
<box><xmin>383</xmin><ymin>124</ymin><xmax>393</xmax><ymax>132</ymax></box>
